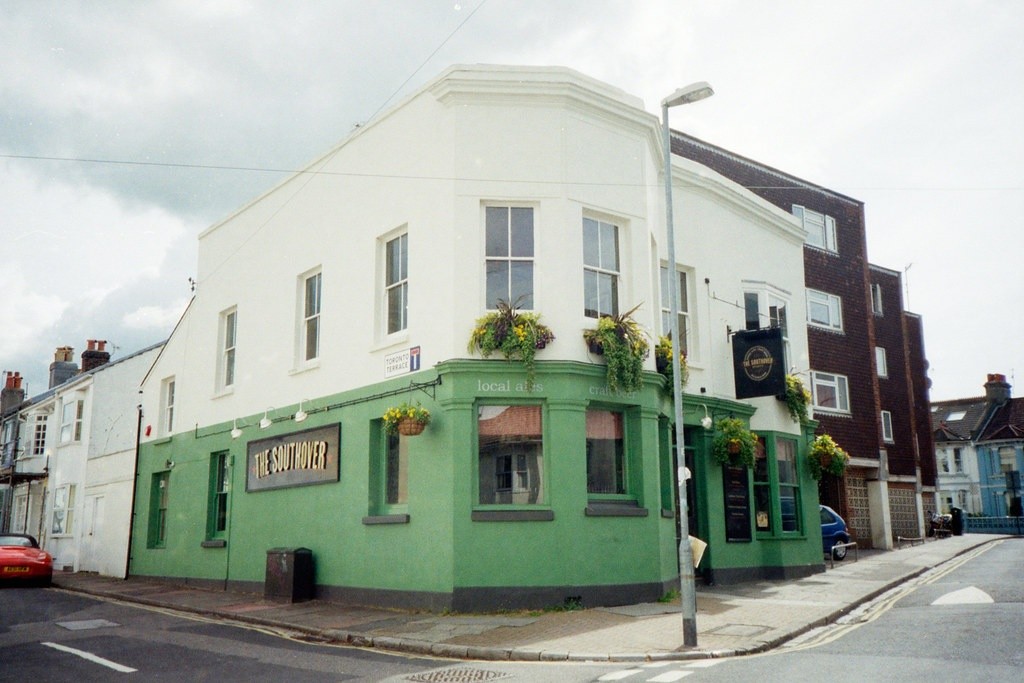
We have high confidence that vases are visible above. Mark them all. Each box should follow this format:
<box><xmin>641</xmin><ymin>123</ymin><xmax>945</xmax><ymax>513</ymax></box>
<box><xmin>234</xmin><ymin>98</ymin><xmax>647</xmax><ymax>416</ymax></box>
<box><xmin>656</xmin><ymin>357</ymin><xmax>669</xmax><ymax>371</ymax></box>
<box><xmin>398</xmin><ymin>417</ymin><xmax>425</xmax><ymax>434</ymax></box>
<box><xmin>589</xmin><ymin>341</ymin><xmax>604</xmax><ymax>355</ymax></box>
<box><xmin>776</xmin><ymin>394</ymin><xmax>788</xmax><ymax>401</ymax></box>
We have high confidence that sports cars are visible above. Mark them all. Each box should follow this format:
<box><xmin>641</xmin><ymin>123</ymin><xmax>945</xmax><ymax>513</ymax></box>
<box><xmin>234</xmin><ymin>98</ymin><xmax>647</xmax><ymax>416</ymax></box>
<box><xmin>0</xmin><ymin>532</ymin><xmax>54</xmax><ymax>589</ymax></box>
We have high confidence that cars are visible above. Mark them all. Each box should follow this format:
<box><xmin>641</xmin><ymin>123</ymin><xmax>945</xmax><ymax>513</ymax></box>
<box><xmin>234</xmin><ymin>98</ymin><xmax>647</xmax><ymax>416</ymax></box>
<box><xmin>780</xmin><ymin>495</ymin><xmax>849</xmax><ymax>560</ymax></box>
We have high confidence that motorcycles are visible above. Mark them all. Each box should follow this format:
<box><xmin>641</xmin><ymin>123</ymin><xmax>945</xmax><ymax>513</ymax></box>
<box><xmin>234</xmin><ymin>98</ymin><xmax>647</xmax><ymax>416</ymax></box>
<box><xmin>926</xmin><ymin>510</ymin><xmax>953</xmax><ymax>538</ymax></box>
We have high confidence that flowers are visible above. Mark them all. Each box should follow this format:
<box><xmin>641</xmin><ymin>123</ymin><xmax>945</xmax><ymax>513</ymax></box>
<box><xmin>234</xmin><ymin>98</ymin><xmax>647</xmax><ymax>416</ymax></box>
<box><xmin>785</xmin><ymin>373</ymin><xmax>813</xmax><ymax>426</ymax></box>
<box><xmin>712</xmin><ymin>411</ymin><xmax>765</xmax><ymax>469</ymax></box>
<box><xmin>656</xmin><ymin>328</ymin><xmax>691</xmax><ymax>395</ymax></box>
<box><xmin>467</xmin><ymin>293</ymin><xmax>556</xmax><ymax>391</ymax></box>
<box><xmin>583</xmin><ymin>299</ymin><xmax>652</xmax><ymax>392</ymax></box>
<box><xmin>381</xmin><ymin>402</ymin><xmax>432</xmax><ymax>438</ymax></box>
<box><xmin>806</xmin><ymin>433</ymin><xmax>850</xmax><ymax>481</ymax></box>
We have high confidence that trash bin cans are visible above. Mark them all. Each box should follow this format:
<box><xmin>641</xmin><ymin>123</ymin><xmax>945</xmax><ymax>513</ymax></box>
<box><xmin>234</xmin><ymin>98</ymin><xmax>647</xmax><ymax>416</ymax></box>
<box><xmin>950</xmin><ymin>507</ymin><xmax>968</xmax><ymax>535</ymax></box>
<box><xmin>262</xmin><ymin>545</ymin><xmax>313</xmax><ymax>605</ymax></box>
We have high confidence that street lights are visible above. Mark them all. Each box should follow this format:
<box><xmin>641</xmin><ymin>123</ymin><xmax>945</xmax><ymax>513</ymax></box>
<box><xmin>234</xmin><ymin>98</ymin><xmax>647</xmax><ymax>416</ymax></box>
<box><xmin>658</xmin><ymin>80</ymin><xmax>717</xmax><ymax>648</ymax></box>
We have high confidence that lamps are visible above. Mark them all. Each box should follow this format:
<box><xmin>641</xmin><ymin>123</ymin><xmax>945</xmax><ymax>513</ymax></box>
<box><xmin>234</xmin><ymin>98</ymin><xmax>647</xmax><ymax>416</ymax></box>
<box><xmin>232</xmin><ymin>415</ymin><xmax>260</xmax><ymax>438</ymax></box>
<box><xmin>260</xmin><ymin>406</ymin><xmax>292</xmax><ymax>430</ymax></box>
<box><xmin>694</xmin><ymin>402</ymin><xmax>712</xmax><ymax>429</ymax></box>
<box><xmin>295</xmin><ymin>398</ymin><xmax>329</xmax><ymax>422</ymax></box>
